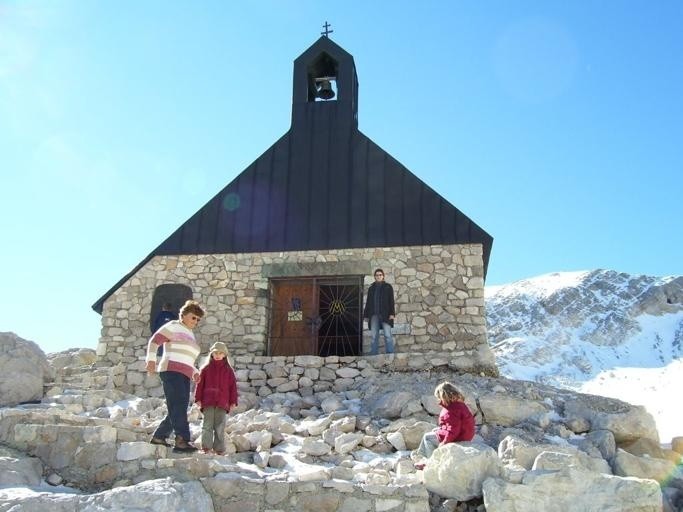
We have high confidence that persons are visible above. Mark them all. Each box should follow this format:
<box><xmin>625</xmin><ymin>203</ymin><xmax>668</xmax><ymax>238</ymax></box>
<box><xmin>414</xmin><ymin>381</ymin><xmax>476</xmax><ymax>473</ymax></box>
<box><xmin>364</xmin><ymin>268</ymin><xmax>395</xmax><ymax>356</ymax></box>
<box><xmin>145</xmin><ymin>300</ymin><xmax>205</xmax><ymax>454</ymax></box>
<box><xmin>194</xmin><ymin>341</ymin><xmax>239</xmax><ymax>456</ymax></box>
<box><xmin>151</xmin><ymin>302</ymin><xmax>179</xmax><ymax>358</ymax></box>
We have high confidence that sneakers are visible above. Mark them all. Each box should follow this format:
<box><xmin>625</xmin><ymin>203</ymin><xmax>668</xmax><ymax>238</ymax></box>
<box><xmin>201</xmin><ymin>448</ymin><xmax>210</xmax><ymax>454</ymax></box>
<box><xmin>413</xmin><ymin>456</ymin><xmax>427</xmax><ymax>470</ymax></box>
<box><xmin>216</xmin><ymin>451</ymin><xmax>224</xmax><ymax>455</ymax></box>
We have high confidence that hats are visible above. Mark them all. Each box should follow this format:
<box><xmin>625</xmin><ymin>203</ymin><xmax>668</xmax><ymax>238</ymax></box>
<box><xmin>210</xmin><ymin>342</ymin><xmax>228</xmax><ymax>356</ymax></box>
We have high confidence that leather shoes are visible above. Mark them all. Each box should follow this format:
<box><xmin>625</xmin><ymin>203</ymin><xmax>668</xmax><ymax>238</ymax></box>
<box><xmin>173</xmin><ymin>446</ymin><xmax>197</xmax><ymax>452</ymax></box>
<box><xmin>150</xmin><ymin>440</ymin><xmax>172</xmax><ymax>447</ymax></box>
